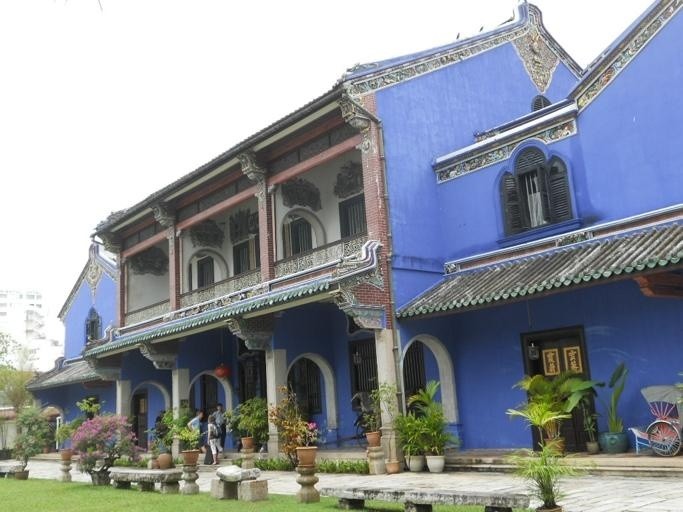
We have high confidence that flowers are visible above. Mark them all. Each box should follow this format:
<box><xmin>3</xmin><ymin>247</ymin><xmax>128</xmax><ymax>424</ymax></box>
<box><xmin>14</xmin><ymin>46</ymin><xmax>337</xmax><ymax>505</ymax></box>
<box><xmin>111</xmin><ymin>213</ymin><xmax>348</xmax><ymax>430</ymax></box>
<box><xmin>269</xmin><ymin>383</ymin><xmax>319</xmax><ymax>456</ymax></box>
<box><xmin>72</xmin><ymin>412</ymin><xmax>146</xmax><ymax>474</ymax></box>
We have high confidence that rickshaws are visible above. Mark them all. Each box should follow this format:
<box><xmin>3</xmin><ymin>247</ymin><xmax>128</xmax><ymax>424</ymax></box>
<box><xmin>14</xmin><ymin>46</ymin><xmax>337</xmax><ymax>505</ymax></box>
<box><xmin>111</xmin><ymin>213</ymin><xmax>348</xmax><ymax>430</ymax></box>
<box><xmin>627</xmin><ymin>384</ymin><xmax>683</xmax><ymax>457</ymax></box>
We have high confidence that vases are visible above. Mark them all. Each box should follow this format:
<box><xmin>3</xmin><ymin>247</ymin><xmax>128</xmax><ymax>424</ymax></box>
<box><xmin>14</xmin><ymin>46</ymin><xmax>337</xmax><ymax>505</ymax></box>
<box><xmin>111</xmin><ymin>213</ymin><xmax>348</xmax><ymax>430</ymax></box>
<box><xmin>87</xmin><ymin>469</ymin><xmax>112</xmax><ymax>485</ymax></box>
<box><xmin>295</xmin><ymin>445</ymin><xmax>318</xmax><ymax>465</ymax></box>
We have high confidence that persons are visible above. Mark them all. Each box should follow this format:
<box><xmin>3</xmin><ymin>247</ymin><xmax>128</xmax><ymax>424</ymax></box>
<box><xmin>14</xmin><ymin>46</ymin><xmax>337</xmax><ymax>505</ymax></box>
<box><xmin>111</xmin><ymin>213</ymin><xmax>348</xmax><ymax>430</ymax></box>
<box><xmin>188</xmin><ymin>410</ymin><xmax>207</xmax><ymax>453</ymax></box>
<box><xmin>207</xmin><ymin>403</ymin><xmax>228</xmax><ymax>464</ymax></box>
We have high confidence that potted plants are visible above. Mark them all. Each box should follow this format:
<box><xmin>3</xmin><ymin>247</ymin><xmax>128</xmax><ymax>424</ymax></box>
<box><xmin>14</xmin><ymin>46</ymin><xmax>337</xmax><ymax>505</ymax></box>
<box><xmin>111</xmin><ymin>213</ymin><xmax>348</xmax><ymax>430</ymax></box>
<box><xmin>147</xmin><ymin>409</ymin><xmax>203</xmax><ymax>468</ymax></box>
<box><xmin>13</xmin><ymin>408</ymin><xmax>51</xmax><ymax>480</ymax></box>
<box><xmin>363</xmin><ymin>379</ymin><xmax>462</xmax><ymax>472</ymax></box>
<box><xmin>55</xmin><ymin>422</ymin><xmax>72</xmax><ymax>461</ymax></box>
<box><xmin>224</xmin><ymin>397</ymin><xmax>269</xmax><ymax>449</ymax></box>
<box><xmin>505</xmin><ymin>360</ymin><xmax>630</xmax><ymax>511</ymax></box>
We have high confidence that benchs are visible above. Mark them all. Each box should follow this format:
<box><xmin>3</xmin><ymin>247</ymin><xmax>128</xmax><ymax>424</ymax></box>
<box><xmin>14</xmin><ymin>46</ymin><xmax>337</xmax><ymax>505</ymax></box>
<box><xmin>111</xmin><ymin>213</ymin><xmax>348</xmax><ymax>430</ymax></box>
<box><xmin>210</xmin><ymin>465</ymin><xmax>268</xmax><ymax>501</ymax></box>
<box><xmin>319</xmin><ymin>476</ymin><xmax>529</xmax><ymax>511</ymax></box>
<box><xmin>112</xmin><ymin>469</ymin><xmax>183</xmax><ymax>492</ymax></box>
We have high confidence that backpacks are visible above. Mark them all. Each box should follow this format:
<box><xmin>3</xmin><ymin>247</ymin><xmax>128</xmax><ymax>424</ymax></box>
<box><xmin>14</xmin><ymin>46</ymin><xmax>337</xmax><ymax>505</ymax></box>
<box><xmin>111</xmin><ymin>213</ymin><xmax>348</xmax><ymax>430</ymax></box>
<box><xmin>211</xmin><ymin>422</ymin><xmax>223</xmax><ymax>438</ymax></box>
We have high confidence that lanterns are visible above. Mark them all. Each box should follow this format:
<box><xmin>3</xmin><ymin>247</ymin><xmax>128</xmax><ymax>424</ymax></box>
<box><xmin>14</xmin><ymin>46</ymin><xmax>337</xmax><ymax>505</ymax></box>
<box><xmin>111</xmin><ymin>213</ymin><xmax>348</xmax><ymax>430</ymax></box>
<box><xmin>216</xmin><ymin>367</ymin><xmax>229</xmax><ymax>378</ymax></box>
